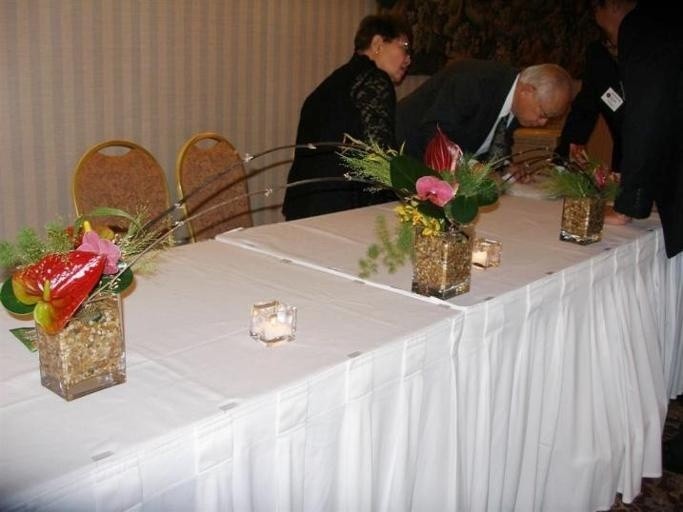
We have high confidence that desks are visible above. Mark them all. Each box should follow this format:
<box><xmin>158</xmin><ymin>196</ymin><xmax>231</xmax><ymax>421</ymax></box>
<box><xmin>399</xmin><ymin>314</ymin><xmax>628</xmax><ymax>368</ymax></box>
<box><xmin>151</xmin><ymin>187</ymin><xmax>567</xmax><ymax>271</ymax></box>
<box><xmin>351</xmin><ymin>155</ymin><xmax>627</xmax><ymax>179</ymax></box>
<box><xmin>1</xmin><ymin>236</ymin><xmax>464</xmax><ymax>511</ymax></box>
<box><xmin>215</xmin><ymin>180</ymin><xmax>681</xmax><ymax>511</ymax></box>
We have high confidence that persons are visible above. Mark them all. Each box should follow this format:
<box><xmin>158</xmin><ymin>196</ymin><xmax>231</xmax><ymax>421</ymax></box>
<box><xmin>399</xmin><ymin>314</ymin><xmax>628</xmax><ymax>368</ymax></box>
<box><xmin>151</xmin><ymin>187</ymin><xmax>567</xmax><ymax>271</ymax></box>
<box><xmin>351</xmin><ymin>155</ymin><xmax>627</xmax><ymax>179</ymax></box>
<box><xmin>552</xmin><ymin>31</ymin><xmax>627</xmax><ymax>171</ymax></box>
<box><xmin>590</xmin><ymin>1</ymin><xmax>683</xmax><ymax>260</ymax></box>
<box><xmin>398</xmin><ymin>56</ymin><xmax>577</xmax><ymax>174</ymax></box>
<box><xmin>280</xmin><ymin>12</ymin><xmax>413</xmax><ymax>222</ymax></box>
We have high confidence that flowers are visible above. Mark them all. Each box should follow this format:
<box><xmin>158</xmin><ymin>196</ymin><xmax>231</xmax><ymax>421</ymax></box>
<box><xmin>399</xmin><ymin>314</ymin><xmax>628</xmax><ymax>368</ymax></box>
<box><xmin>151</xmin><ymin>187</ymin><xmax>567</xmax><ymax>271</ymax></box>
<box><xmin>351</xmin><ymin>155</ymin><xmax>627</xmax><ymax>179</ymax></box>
<box><xmin>557</xmin><ymin>142</ymin><xmax>620</xmax><ymax>195</ymax></box>
<box><xmin>385</xmin><ymin>131</ymin><xmax>496</xmax><ymax>230</ymax></box>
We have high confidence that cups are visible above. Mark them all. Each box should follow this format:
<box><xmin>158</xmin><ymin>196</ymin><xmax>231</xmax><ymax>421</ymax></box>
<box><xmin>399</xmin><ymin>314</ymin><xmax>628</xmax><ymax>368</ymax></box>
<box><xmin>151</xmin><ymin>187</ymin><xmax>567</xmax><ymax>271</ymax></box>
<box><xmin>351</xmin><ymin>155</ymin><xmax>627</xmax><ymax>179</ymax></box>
<box><xmin>473</xmin><ymin>237</ymin><xmax>502</xmax><ymax>268</ymax></box>
<box><xmin>250</xmin><ymin>299</ymin><xmax>296</xmax><ymax>343</ymax></box>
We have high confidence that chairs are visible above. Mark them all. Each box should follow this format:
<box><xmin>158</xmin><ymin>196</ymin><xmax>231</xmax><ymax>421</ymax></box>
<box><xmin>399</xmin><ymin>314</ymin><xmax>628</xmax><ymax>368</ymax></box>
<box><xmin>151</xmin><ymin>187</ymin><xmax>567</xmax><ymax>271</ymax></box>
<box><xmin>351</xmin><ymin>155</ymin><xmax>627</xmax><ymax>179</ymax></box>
<box><xmin>70</xmin><ymin>132</ymin><xmax>254</xmax><ymax>252</ymax></box>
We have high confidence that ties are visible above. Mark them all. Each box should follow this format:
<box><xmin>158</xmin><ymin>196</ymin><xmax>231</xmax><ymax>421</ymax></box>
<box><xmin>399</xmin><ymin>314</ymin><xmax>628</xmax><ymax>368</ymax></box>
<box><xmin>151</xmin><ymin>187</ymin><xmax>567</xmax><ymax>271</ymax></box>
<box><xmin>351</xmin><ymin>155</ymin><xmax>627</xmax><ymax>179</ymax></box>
<box><xmin>488</xmin><ymin>113</ymin><xmax>511</xmax><ymax>161</ymax></box>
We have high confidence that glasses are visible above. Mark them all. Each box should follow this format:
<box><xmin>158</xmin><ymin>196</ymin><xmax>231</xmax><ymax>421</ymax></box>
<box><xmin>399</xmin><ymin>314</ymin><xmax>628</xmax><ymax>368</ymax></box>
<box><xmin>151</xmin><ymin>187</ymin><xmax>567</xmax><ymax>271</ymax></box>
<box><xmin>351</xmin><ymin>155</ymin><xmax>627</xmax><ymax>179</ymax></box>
<box><xmin>382</xmin><ymin>34</ymin><xmax>411</xmax><ymax>51</ymax></box>
